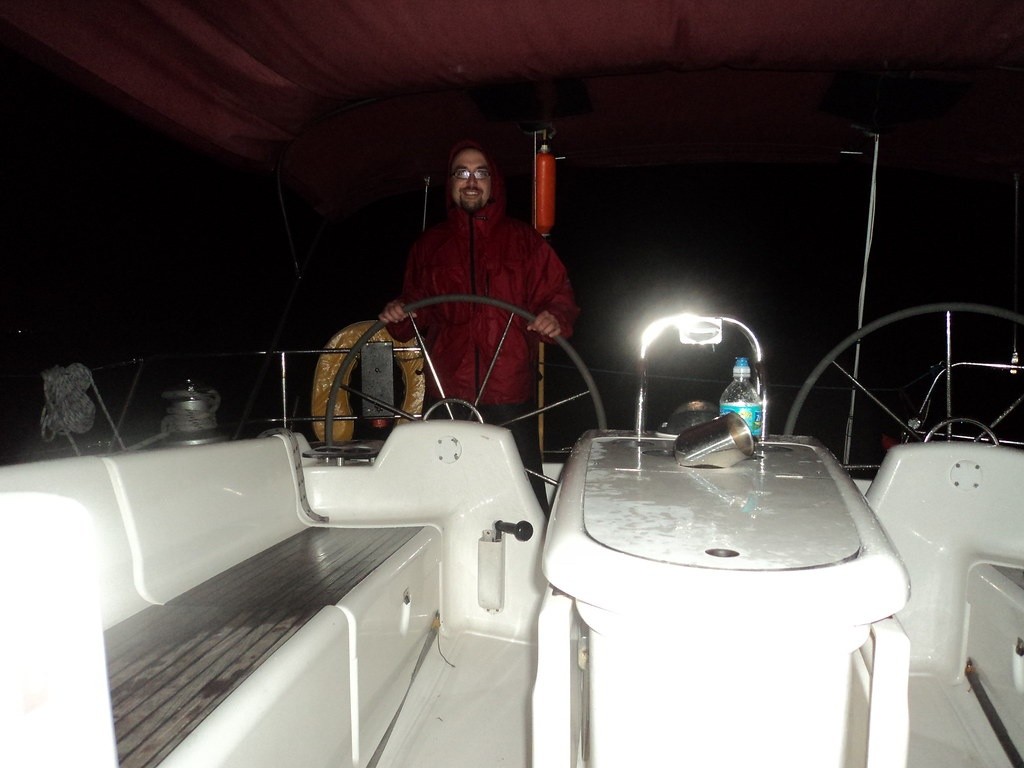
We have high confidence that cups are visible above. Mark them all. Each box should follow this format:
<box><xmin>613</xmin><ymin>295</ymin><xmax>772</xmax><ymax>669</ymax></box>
<box><xmin>673</xmin><ymin>412</ymin><xmax>754</xmax><ymax>468</ymax></box>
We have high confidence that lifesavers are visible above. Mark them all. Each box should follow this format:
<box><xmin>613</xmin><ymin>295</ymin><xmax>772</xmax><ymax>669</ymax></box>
<box><xmin>310</xmin><ymin>317</ymin><xmax>428</xmax><ymax>445</ymax></box>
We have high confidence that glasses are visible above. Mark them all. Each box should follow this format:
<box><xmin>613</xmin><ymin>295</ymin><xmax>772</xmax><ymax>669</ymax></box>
<box><xmin>450</xmin><ymin>169</ymin><xmax>491</xmax><ymax>179</ymax></box>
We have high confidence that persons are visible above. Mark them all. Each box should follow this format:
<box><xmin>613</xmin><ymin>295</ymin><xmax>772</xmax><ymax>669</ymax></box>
<box><xmin>378</xmin><ymin>139</ymin><xmax>581</xmax><ymax>518</ymax></box>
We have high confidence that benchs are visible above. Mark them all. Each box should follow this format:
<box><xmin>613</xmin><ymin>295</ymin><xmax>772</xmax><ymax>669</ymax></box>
<box><xmin>936</xmin><ymin>562</ymin><xmax>1024</xmax><ymax>768</ymax></box>
<box><xmin>1</xmin><ymin>429</ymin><xmax>440</xmax><ymax>768</ymax></box>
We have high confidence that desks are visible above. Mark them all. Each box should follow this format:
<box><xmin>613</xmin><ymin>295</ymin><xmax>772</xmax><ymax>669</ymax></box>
<box><xmin>529</xmin><ymin>430</ymin><xmax>912</xmax><ymax>767</ymax></box>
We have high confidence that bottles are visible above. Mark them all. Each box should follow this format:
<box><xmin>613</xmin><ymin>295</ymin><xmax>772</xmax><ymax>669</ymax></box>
<box><xmin>718</xmin><ymin>357</ymin><xmax>764</xmax><ymax>443</ymax></box>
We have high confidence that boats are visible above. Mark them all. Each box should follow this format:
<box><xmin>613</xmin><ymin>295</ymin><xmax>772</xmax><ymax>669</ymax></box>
<box><xmin>0</xmin><ymin>131</ymin><xmax>1024</xmax><ymax>768</ymax></box>
